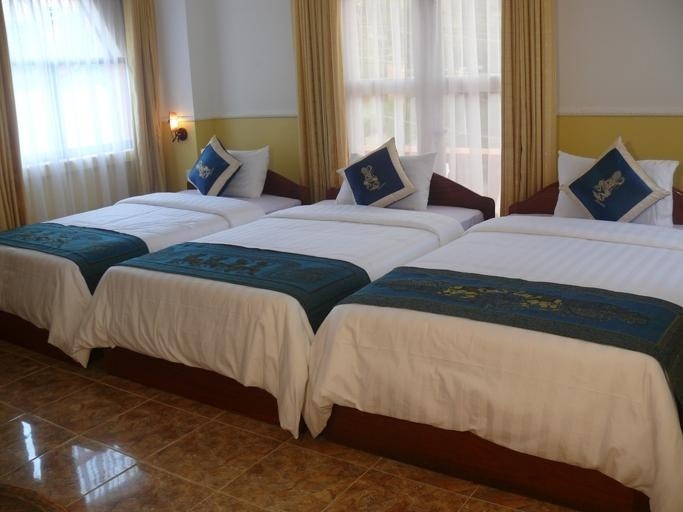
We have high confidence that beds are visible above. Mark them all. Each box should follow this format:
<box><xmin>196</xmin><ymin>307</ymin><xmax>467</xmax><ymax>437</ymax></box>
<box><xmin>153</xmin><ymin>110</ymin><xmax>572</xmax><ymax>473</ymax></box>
<box><xmin>3</xmin><ymin>172</ymin><xmax>309</xmax><ymax>370</ymax></box>
<box><xmin>303</xmin><ymin>172</ymin><xmax>683</xmax><ymax>506</ymax></box>
<box><xmin>70</xmin><ymin>171</ymin><xmax>496</xmax><ymax>438</ymax></box>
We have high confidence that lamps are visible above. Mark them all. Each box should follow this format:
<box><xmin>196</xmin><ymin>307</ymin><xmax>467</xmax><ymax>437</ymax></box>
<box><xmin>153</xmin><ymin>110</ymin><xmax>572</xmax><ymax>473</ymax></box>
<box><xmin>167</xmin><ymin>111</ymin><xmax>187</xmax><ymax>144</ymax></box>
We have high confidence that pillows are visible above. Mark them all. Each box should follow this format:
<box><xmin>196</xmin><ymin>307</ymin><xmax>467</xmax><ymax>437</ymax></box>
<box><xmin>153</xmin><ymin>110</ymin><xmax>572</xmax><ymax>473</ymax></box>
<box><xmin>551</xmin><ymin>135</ymin><xmax>679</xmax><ymax>230</ymax></box>
<box><xmin>185</xmin><ymin>134</ymin><xmax>270</xmax><ymax>201</ymax></box>
<box><xmin>334</xmin><ymin>136</ymin><xmax>438</xmax><ymax>213</ymax></box>
